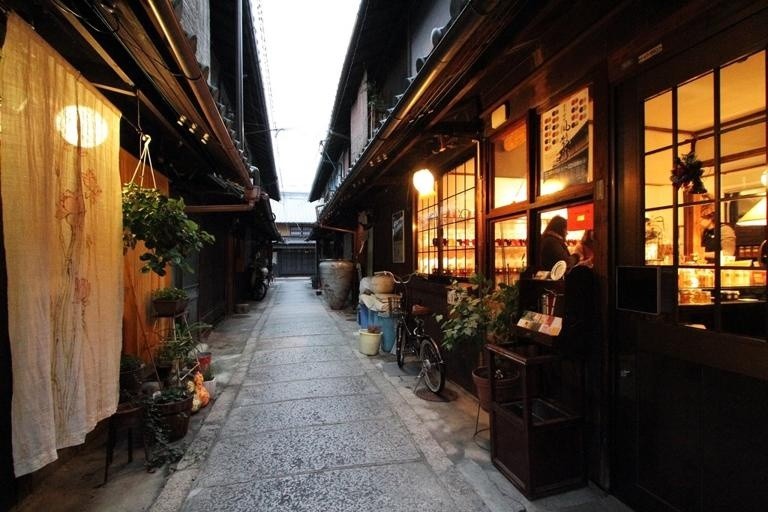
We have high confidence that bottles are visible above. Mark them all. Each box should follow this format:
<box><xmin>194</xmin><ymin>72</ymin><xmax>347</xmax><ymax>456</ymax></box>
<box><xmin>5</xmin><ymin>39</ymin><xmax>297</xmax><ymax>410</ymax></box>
<box><xmin>738</xmin><ymin>245</ymin><xmax>760</xmax><ymax>257</ymax></box>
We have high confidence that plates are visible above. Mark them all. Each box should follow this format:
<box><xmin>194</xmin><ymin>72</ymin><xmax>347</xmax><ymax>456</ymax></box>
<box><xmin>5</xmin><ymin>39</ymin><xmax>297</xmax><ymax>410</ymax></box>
<box><xmin>550</xmin><ymin>259</ymin><xmax>568</xmax><ymax>282</ymax></box>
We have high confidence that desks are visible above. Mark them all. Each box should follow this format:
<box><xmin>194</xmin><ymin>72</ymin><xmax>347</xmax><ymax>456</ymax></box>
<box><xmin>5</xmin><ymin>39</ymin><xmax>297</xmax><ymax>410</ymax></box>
<box><xmin>99</xmin><ymin>404</ymin><xmax>141</xmax><ymax>484</ymax></box>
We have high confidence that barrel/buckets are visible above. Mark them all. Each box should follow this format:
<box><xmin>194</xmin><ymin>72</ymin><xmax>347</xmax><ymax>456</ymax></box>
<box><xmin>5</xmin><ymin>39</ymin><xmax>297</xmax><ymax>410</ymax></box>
<box><xmin>154</xmin><ymin>393</ymin><xmax>194</xmax><ymax>442</ymax></box>
<box><xmin>358</xmin><ymin>328</ymin><xmax>382</xmax><ymax>355</ymax></box>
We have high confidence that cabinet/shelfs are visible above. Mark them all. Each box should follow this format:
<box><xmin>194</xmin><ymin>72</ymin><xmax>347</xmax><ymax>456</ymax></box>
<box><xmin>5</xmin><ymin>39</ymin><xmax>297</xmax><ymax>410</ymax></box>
<box><xmin>482</xmin><ymin>262</ymin><xmax>601</xmax><ymax>500</ymax></box>
<box><xmin>144</xmin><ymin>312</ymin><xmax>198</xmax><ymax>385</ymax></box>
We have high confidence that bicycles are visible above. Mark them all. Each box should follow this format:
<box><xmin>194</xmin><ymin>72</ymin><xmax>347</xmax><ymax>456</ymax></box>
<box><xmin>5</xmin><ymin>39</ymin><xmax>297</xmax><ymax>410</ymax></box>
<box><xmin>248</xmin><ymin>262</ymin><xmax>272</xmax><ymax>302</ymax></box>
<box><xmin>373</xmin><ymin>270</ymin><xmax>447</xmax><ymax>395</ymax></box>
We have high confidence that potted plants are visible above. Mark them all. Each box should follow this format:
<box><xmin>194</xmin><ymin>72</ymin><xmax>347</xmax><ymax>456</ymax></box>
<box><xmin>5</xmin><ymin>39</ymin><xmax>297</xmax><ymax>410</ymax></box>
<box><xmin>153</xmin><ymin>286</ymin><xmax>190</xmax><ymax>315</ymax></box>
<box><xmin>143</xmin><ymin>385</ymin><xmax>195</xmax><ymax>470</ymax></box>
<box><xmin>201</xmin><ymin>363</ymin><xmax>218</xmax><ymax>401</ymax></box>
<box><xmin>431</xmin><ymin>269</ymin><xmax>521</xmax><ymax>409</ymax></box>
<box><xmin>357</xmin><ymin>326</ymin><xmax>384</xmax><ymax>356</ymax></box>
<box><xmin>119</xmin><ymin>356</ymin><xmax>149</xmax><ymax>402</ymax></box>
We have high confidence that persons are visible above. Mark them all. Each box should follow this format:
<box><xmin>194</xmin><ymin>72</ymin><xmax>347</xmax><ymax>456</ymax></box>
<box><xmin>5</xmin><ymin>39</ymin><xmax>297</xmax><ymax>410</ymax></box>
<box><xmin>700</xmin><ymin>205</ymin><xmax>736</xmax><ymax>265</ymax></box>
<box><xmin>572</xmin><ymin>229</ymin><xmax>592</xmax><ymax>267</ymax></box>
<box><xmin>541</xmin><ymin>215</ymin><xmax>580</xmax><ymax>271</ymax></box>
<box><xmin>645</xmin><ymin>218</ymin><xmax>658</xmax><ymax>241</ymax></box>
